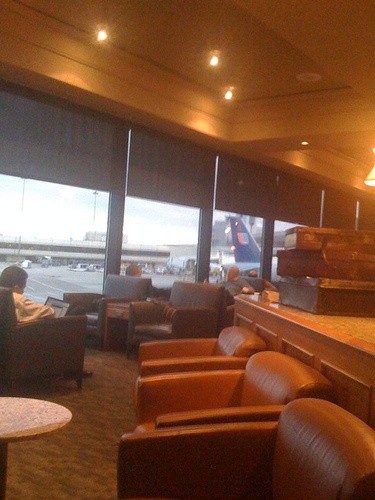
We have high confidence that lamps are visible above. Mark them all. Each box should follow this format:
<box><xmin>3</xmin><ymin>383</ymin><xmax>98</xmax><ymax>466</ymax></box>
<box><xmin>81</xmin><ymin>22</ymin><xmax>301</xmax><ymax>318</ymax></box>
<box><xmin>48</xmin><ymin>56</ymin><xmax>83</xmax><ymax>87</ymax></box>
<box><xmin>364</xmin><ymin>165</ymin><xmax>375</xmax><ymax>186</ymax></box>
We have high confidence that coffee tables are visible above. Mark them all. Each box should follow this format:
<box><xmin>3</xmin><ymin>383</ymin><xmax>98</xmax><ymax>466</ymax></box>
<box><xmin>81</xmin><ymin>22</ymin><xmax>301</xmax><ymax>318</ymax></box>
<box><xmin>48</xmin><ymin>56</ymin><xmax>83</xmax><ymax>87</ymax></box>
<box><xmin>105</xmin><ymin>299</ymin><xmax>168</xmax><ymax>351</ymax></box>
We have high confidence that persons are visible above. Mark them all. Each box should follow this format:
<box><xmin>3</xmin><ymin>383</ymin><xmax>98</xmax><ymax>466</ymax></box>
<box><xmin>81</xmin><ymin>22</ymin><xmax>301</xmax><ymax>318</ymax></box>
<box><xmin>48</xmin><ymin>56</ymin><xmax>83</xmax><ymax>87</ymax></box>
<box><xmin>248</xmin><ymin>270</ymin><xmax>276</xmax><ymax>290</ymax></box>
<box><xmin>126</xmin><ymin>264</ymin><xmax>142</xmax><ymax>277</ymax></box>
<box><xmin>0</xmin><ymin>265</ymin><xmax>91</xmax><ymax>378</ymax></box>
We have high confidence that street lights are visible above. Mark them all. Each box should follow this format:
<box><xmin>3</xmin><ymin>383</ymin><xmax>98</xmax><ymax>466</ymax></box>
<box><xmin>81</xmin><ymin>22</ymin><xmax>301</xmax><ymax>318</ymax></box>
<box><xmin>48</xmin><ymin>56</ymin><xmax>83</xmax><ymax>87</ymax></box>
<box><xmin>92</xmin><ymin>191</ymin><xmax>99</xmax><ymax>232</ymax></box>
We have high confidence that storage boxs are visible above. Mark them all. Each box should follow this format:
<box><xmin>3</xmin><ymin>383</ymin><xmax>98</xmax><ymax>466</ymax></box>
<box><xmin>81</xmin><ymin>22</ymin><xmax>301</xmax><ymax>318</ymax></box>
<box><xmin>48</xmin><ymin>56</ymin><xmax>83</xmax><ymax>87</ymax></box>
<box><xmin>285</xmin><ymin>227</ymin><xmax>375</xmax><ymax>252</ymax></box>
<box><xmin>276</xmin><ymin>247</ymin><xmax>375</xmax><ymax>281</ymax></box>
<box><xmin>278</xmin><ymin>275</ymin><xmax>375</xmax><ymax>318</ymax></box>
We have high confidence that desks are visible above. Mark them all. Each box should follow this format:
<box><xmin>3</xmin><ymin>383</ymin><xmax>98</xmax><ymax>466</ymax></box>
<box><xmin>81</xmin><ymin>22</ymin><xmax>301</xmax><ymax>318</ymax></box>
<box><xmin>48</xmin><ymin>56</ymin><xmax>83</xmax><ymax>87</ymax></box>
<box><xmin>232</xmin><ymin>293</ymin><xmax>375</xmax><ymax>430</ymax></box>
<box><xmin>0</xmin><ymin>397</ymin><xmax>73</xmax><ymax>500</ymax></box>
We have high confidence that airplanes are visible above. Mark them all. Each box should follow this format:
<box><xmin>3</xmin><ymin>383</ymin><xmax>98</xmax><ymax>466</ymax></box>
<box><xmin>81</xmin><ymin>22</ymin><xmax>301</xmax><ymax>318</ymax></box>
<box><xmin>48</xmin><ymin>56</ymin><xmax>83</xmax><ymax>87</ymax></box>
<box><xmin>221</xmin><ymin>217</ymin><xmax>281</xmax><ymax>282</ymax></box>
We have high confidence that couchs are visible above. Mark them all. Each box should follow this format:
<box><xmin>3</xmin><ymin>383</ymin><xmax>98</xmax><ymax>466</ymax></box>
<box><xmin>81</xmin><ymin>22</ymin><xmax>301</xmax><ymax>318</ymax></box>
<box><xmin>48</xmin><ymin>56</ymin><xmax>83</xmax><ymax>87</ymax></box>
<box><xmin>63</xmin><ymin>273</ymin><xmax>152</xmax><ymax>352</ymax></box>
<box><xmin>134</xmin><ymin>349</ymin><xmax>333</xmax><ymax>435</ymax></box>
<box><xmin>138</xmin><ymin>325</ymin><xmax>267</xmax><ymax>381</ymax></box>
<box><xmin>115</xmin><ymin>397</ymin><xmax>375</xmax><ymax>500</ymax></box>
<box><xmin>0</xmin><ymin>286</ymin><xmax>88</xmax><ymax>390</ymax></box>
<box><xmin>125</xmin><ymin>281</ymin><xmax>224</xmax><ymax>362</ymax></box>
